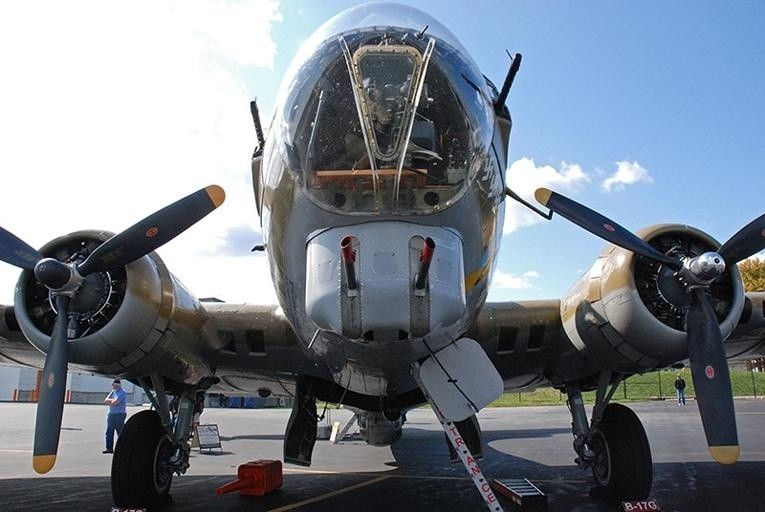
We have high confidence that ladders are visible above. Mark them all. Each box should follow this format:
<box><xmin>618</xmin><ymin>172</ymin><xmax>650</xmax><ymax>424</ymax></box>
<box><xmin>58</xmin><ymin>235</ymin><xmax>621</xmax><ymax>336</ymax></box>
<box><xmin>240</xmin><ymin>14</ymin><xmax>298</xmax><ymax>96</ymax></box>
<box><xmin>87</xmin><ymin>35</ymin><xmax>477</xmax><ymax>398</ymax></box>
<box><xmin>488</xmin><ymin>477</ymin><xmax>548</xmax><ymax>507</ymax></box>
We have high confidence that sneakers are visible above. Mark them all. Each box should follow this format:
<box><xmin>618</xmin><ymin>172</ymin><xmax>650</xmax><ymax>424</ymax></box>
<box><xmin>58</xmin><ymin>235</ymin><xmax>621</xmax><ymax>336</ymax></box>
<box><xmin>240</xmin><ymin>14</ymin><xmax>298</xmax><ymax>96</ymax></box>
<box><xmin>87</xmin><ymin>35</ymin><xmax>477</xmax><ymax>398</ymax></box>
<box><xmin>103</xmin><ymin>449</ymin><xmax>113</xmax><ymax>454</ymax></box>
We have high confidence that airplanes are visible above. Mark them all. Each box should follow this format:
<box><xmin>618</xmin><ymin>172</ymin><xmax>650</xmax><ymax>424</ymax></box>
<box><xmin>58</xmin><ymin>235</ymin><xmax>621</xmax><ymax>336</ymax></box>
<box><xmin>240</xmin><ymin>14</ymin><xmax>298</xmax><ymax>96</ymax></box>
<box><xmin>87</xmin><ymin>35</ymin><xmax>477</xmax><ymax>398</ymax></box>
<box><xmin>0</xmin><ymin>0</ymin><xmax>765</xmax><ymax>502</ymax></box>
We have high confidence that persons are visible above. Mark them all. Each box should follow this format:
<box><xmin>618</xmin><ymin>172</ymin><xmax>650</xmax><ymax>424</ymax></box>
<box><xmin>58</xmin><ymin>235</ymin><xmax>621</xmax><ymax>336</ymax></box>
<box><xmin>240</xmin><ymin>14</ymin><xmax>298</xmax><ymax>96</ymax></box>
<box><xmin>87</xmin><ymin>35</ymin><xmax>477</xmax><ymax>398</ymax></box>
<box><xmin>675</xmin><ymin>374</ymin><xmax>685</xmax><ymax>406</ymax></box>
<box><xmin>102</xmin><ymin>380</ymin><xmax>127</xmax><ymax>454</ymax></box>
